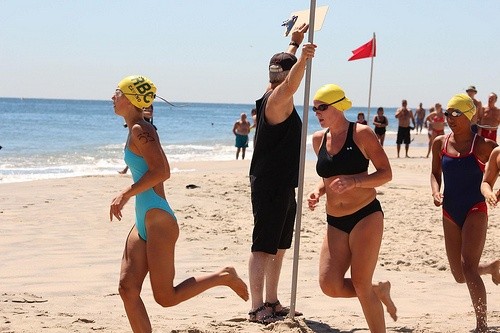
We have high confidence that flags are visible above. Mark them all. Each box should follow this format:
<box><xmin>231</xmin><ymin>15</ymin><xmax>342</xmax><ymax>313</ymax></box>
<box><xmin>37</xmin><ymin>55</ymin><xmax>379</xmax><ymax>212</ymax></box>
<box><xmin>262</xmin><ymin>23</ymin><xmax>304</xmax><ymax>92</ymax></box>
<box><xmin>347</xmin><ymin>37</ymin><xmax>377</xmax><ymax>61</ymax></box>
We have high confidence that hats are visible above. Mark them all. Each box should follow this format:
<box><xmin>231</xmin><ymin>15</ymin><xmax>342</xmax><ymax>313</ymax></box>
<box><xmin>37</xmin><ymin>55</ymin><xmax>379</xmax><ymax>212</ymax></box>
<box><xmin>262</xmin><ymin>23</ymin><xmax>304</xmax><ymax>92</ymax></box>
<box><xmin>118</xmin><ymin>75</ymin><xmax>157</xmax><ymax>109</ymax></box>
<box><xmin>269</xmin><ymin>52</ymin><xmax>297</xmax><ymax>72</ymax></box>
<box><xmin>314</xmin><ymin>83</ymin><xmax>352</xmax><ymax>112</ymax></box>
<box><xmin>446</xmin><ymin>93</ymin><xmax>477</xmax><ymax>122</ymax></box>
<box><xmin>466</xmin><ymin>86</ymin><xmax>477</xmax><ymax>94</ymax></box>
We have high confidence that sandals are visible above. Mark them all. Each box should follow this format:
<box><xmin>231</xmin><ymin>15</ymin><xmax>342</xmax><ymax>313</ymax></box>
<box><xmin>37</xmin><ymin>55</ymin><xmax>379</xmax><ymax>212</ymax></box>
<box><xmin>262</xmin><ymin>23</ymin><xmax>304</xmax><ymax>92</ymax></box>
<box><xmin>248</xmin><ymin>307</ymin><xmax>286</xmax><ymax>326</ymax></box>
<box><xmin>263</xmin><ymin>300</ymin><xmax>303</xmax><ymax>316</ymax></box>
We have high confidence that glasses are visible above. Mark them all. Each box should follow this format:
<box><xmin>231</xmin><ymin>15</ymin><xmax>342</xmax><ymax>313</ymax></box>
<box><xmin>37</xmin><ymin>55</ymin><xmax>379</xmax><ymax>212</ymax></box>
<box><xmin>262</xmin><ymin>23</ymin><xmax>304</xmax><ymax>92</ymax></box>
<box><xmin>444</xmin><ymin>111</ymin><xmax>463</xmax><ymax>117</ymax></box>
<box><xmin>313</xmin><ymin>104</ymin><xmax>328</xmax><ymax>112</ymax></box>
<box><xmin>114</xmin><ymin>90</ymin><xmax>123</xmax><ymax>98</ymax></box>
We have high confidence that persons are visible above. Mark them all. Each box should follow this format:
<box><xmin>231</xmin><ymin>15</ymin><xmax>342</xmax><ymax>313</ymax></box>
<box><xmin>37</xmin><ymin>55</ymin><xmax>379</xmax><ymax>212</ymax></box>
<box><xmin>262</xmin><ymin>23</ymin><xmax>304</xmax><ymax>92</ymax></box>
<box><xmin>480</xmin><ymin>146</ymin><xmax>500</xmax><ymax>208</ymax></box>
<box><xmin>394</xmin><ymin>99</ymin><xmax>416</xmax><ymax>159</ymax></box>
<box><xmin>233</xmin><ymin>113</ymin><xmax>251</xmax><ymax>161</ymax></box>
<box><xmin>308</xmin><ymin>84</ymin><xmax>398</xmax><ymax>333</ymax></box>
<box><xmin>248</xmin><ymin>109</ymin><xmax>257</xmax><ymax>130</ymax></box>
<box><xmin>424</xmin><ymin>103</ymin><xmax>446</xmax><ymax>159</ymax></box>
<box><xmin>413</xmin><ymin>102</ymin><xmax>426</xmax><ymax>135</ymax></box>
<box><xmin>373</xmin><ymin>106</ymin><xmax>388</xmax><ymax>146</ymax></box>
<box><xmin>429</xmin><ymin>93</ymin><xmax>500</xmax><ymax>333</ymax></box>
<box><xmin>246</xmin><ymin>23</ymin><xmax>318</xmax><ymax>326</ymax></box>
<box><xmin>465</xmin><ymin>85</ymin><xmax>500</xmax><ymax>140</ymax></box>
<box><xmin>356</xmin><ymin>112</ymin><xmax>367</xmax><ymax>125</ymax></box>
<box><xmin>109</xmin><ymin>75</ymin><xmax>249</xmax><ymax>333</ymax></box>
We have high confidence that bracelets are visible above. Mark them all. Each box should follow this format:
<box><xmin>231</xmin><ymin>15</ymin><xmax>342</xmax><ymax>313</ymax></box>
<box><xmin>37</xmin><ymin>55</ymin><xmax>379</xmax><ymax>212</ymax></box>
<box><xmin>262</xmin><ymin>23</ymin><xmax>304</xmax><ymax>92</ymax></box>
<box><xmin>289</xmin><ymin>42</ymin><xmax>299</xmax><ymax>48</ymax></box>
